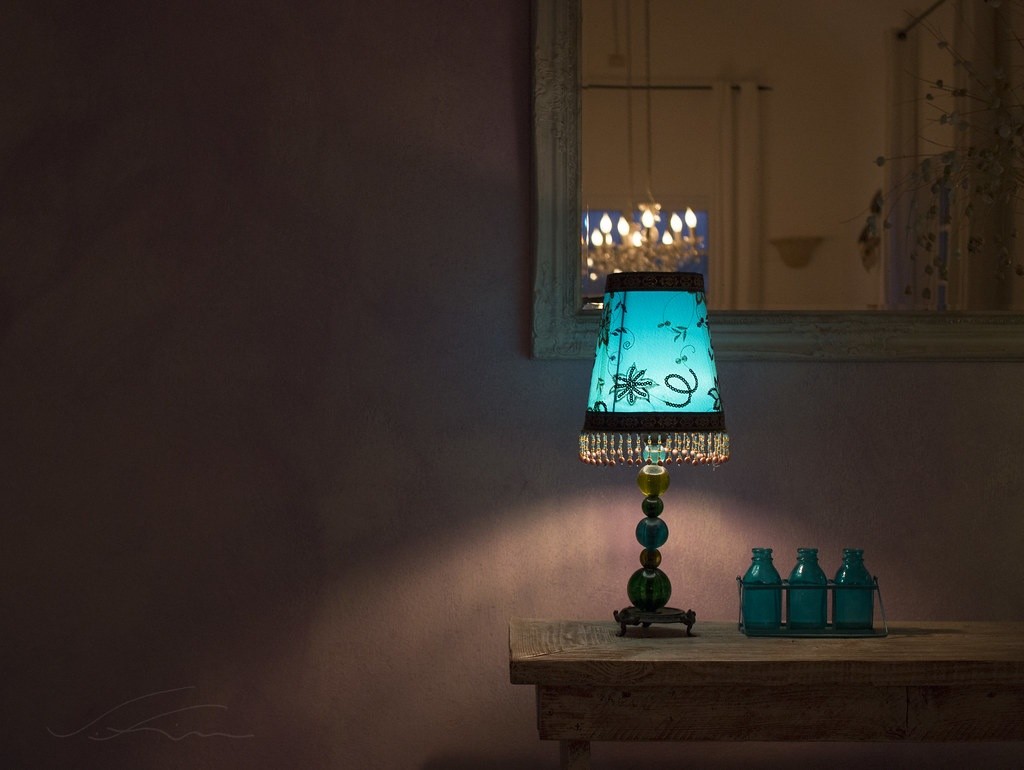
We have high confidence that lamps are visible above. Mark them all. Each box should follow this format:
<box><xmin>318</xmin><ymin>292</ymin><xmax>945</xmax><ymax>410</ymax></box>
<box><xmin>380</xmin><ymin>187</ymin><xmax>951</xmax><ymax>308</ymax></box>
<box><xmin>588</xmin><ymin>0</ymin><xmax>704</xmax><ymax>282</ymax></box>
<box><xmin>579</xmin><ymin>271</ymin><xmax>735</xmax><ymax>637</ymax></box>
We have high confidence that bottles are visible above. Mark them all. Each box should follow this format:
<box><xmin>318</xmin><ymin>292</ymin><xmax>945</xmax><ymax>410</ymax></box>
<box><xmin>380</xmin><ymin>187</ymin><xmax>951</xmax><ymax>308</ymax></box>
<box><xmin>785</xmin><ymin>547</ymin><xmax>828</xmax><ymax>630</ymax></box>
<box><xmin>741</xmin><ymin>548</ymin><xmax>782</xmax><ymax>632</ymax></box>
<box><xmin>832</xmin><ymin>548</ymin><xmax>874</xmax><ymax>631</ymax></box>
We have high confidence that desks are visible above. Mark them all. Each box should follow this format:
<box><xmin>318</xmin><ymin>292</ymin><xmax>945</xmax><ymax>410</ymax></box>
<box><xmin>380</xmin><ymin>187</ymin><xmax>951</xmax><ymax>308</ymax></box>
<box><xmin>509</xmin><ymin>621</ymin><xmax>1024</xmax><ymax>770</ymax></box>
